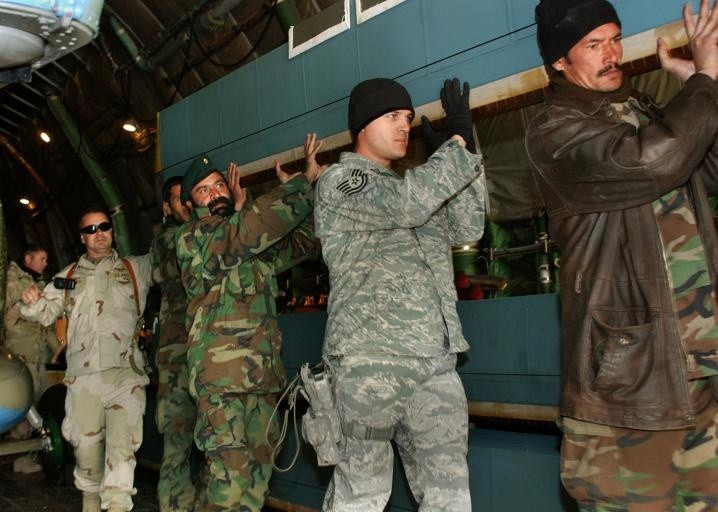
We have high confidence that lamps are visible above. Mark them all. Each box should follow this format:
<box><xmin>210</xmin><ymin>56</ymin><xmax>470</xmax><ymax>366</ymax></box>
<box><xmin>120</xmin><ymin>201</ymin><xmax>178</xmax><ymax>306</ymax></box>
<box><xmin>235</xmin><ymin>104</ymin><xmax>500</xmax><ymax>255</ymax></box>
<box><xmin>122</xmin><ymin>115</ymin><xmax>155</xmax><ymax>151</ymax></box>
<box><xmin>19</xmin><ymin>197</ymin><xmax>40</xmax><ymax>217</ymax></box>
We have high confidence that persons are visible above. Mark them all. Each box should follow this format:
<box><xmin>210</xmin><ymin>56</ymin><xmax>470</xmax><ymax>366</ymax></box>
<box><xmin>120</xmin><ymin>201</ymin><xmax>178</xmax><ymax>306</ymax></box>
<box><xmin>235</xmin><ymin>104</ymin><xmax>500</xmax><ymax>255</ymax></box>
<box><xmin>525</xmin><ymin>0</ymin><xmax>718</xmax><ymax>512</ymax></box>
<box><xmin>4</xmin><ymin>245</ymin><xmax>61</xmax><ymax>478</ymax></box>
<box><xmin>20</xmin><ymin>211</ymin><xmax>164</xmax><ymax>512</ymax></box>
<box><xmin>175</xmin><ymin>129</ymin><xmax>329</xmax><ymax>512</ymax></box>
<box><xmin>300</xmin><ymin>77</ymin><xmax>487</xmax><ymax>512</ymax></box>
<box><xmin>150</xmin><ymin>162</ymin><xmax>246</xmax><ymax>512</ymax></box>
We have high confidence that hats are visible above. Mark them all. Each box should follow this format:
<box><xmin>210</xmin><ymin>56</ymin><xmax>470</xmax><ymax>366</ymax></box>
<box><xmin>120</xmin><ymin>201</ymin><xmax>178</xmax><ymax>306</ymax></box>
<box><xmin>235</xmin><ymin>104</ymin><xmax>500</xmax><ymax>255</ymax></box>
<box><xmin>179</xmin><ymin>154</ymin><xmax>218</xmax><ymax>206</ymax></box>
<box><xmin>533</xmin><ymin>0</ymin><xmax>621</xmax><ymax>66</ymax></box>
<box><xmin>347</xmin><ymin>76</ymin><xmax>415</xmax><ymax>142</ymax></box>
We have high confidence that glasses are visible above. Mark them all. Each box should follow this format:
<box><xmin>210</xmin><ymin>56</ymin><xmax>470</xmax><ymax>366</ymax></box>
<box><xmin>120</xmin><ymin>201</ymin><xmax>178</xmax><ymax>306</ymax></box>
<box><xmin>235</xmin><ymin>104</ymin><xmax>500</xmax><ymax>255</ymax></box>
<box><xmin>77</xmin><ymin>221</ymin><xmax>112</xmax><ymax>235</ymax></box>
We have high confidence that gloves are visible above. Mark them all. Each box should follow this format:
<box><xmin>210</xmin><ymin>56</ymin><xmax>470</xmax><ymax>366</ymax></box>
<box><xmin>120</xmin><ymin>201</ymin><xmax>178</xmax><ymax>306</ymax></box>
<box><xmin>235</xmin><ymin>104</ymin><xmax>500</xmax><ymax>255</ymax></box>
<box><xmin>437</xmin><ymin>80</ymin><xmax>473</xmax><ymax>142</ymax></box>
<box><xmin>419</xmin><ymin>115</ymin><xmax>440</xmax><ymax>161</ymax></box>
